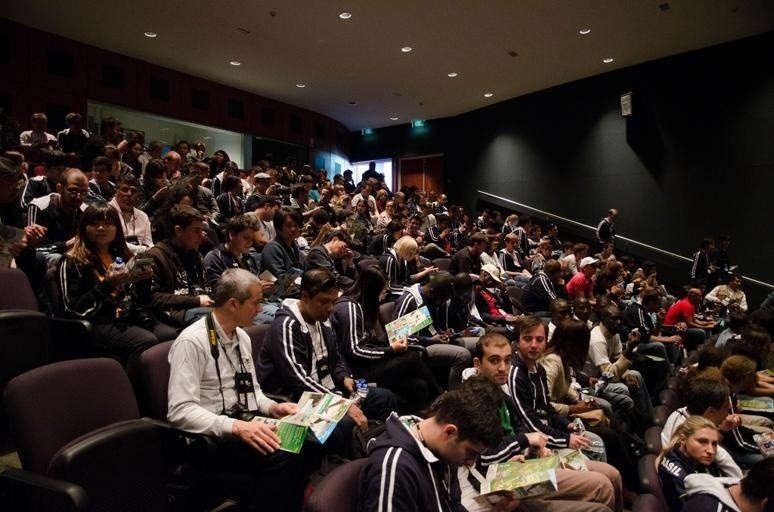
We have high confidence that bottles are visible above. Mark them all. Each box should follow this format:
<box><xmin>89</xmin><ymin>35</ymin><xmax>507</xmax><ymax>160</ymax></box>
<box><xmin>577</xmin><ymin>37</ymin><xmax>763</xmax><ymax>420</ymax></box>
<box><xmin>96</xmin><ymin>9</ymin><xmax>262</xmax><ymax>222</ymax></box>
<box><xmin>112</xmin><ymin>256</ymin><xmax>125</xmax><ymax>274</ymax></box>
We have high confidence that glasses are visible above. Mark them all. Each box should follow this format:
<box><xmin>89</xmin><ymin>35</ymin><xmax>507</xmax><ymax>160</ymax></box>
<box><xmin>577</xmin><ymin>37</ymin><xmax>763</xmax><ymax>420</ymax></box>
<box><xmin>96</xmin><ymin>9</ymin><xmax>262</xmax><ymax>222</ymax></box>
<box><xmin>317</xmin><ymin>277</ymin><xmax>341</xmax><ymax>293</ymax></box>
<box><xmin>66</xmin><ymin>190</ymin><xmax>87</xmax><ymax>200</ymax></box>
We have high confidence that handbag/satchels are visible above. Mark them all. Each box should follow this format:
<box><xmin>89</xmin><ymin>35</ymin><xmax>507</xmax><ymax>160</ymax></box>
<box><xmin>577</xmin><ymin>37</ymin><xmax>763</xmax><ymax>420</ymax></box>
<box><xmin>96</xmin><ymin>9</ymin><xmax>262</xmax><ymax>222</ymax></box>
<box><xmin>352</xmin><ymin>420</ymin><xmax>386</xmax><ymax>456</ymax></box>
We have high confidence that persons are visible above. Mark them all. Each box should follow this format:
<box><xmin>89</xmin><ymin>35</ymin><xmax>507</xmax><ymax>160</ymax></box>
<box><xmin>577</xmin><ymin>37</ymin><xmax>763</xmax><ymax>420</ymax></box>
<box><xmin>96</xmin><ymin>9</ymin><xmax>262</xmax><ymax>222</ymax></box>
<box><xmin>0</xmin><ymin>113</ymin><xmax>774</xmax><ymax>512</ymax></box>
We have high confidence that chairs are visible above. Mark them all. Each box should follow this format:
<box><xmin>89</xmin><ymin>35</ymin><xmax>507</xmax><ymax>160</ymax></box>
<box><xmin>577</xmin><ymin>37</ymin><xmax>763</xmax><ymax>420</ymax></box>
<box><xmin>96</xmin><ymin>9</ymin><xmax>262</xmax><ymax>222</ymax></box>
<box><xmin>1</xmin><ymin>268</ymin><xmax>89</xmax><ymax>383</ymax></box>
<box><xmin>243</xmin><ymin>323</ymin><xmax>270</xmax><ymax>367</ymax></box>
<box><xmin>139</xmin><ymin>339</ymin><xmax>180</xmax><ymax>423</ymax></box>
<box><xmin>507</xmin><ymin>284</ymin><xmax>523</xmax><ymax>301</ymax></box>
<box><xmin>1</xmin><ymin>358</ymin><xmax>227</xmax><ymax>512</ymax></box>
<box><xmin>380</xmin><ymin>302</ymin><xmax>396</xmax><ymax>327</ymax></box>
<box><xmin>632</xmin><ymin>330</ymin><xmax>723</xmax><ymax>512</ymax></box>
<box><xmin>306</xmin><ymin>458</ymin><xmax>369</xmax><ymax>511</ymax></box>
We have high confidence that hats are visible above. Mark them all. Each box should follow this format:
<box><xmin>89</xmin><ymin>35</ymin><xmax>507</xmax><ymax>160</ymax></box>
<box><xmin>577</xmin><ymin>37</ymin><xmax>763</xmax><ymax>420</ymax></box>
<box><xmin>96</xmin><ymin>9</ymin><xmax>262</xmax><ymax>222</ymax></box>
<box><xmin>481</xmin><ymin>263</ymin><xmax>502</xmax><ymax>283</ymax></box>
<box><xmin>254</xmin><ymin>173</ymin><xmax>272</xmax><ymax>183</ymax></box>
<box><xmin>579</xmin><ymin>256</ymin><xmax>600</xmax><ymax>268</ymax></box>
